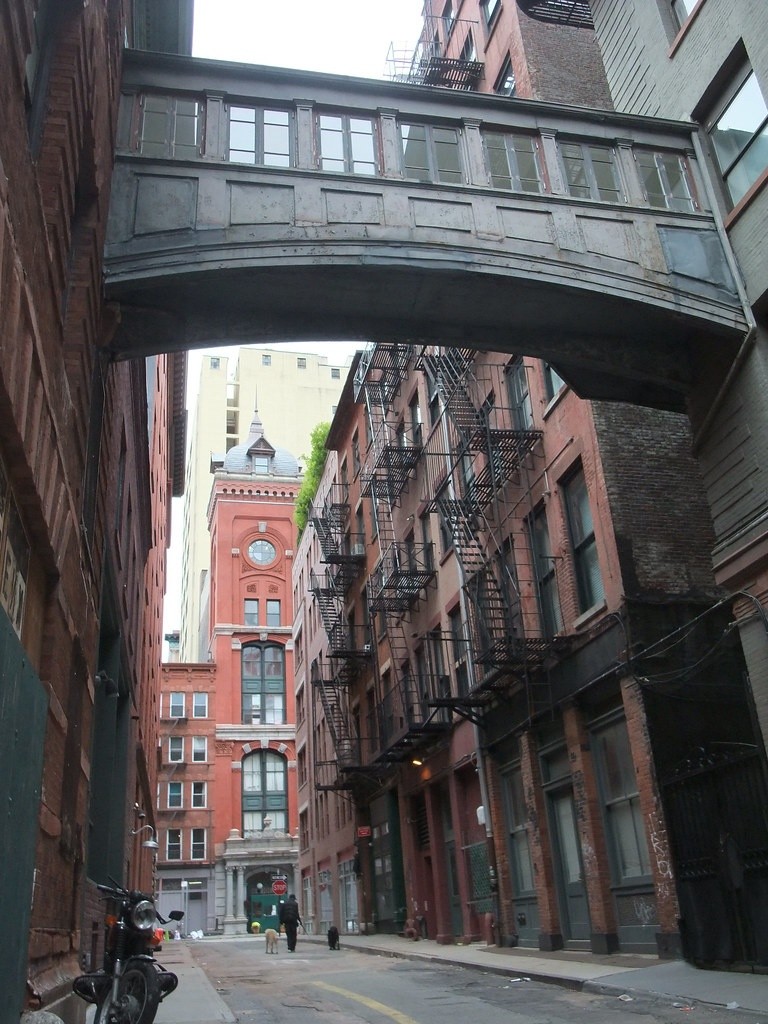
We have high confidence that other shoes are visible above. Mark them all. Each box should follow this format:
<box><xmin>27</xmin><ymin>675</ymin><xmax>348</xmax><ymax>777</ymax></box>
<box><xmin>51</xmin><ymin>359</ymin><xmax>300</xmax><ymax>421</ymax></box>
<box><xmin>287</xmin><ymin>948</ymin><xmax>295</xmax><ymax>953</ymax></box>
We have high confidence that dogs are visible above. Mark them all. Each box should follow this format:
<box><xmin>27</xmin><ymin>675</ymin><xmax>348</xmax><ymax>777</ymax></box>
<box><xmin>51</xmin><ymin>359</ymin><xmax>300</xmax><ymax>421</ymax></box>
<box><xmin>327</xmin><ymin>926</ymin><xmax>340</xmax><ymax>950</ymax></box>
<box><xmin>264</xmin><ymin>929</ymin><xmax>279</xmax><ymax>954</ymax></box>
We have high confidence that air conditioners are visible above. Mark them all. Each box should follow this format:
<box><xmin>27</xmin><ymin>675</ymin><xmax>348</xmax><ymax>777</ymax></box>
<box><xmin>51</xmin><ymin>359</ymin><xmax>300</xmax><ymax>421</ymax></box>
<box><xmin>397</xmin><ymin>578</ymin><xmax>418</xmax><ymax>598</ymax></box>
<box><xmin>351</xmin><ymin>544</ymin><xmax>366</xmax><ymax>559</ymax></box>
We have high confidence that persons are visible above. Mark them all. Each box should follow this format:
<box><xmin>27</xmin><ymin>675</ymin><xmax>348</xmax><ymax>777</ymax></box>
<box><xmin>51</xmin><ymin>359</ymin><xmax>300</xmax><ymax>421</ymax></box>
<box><xmin>279</xmin><ymin>894</ymin><xmax>303</xmax><ymax>952</ymax></box>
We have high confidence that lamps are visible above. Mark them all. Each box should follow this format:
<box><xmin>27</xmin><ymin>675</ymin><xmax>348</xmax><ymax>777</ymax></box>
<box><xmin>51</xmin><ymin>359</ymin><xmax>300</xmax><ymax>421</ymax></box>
<box><xmin>95</xmin><ymin>670</ymin><xmax>119</xmax><ymax>700</ymax></box>
<box><xmin>134</xmin><ymin>802</ymin><xmax>146</xmax><ymax>819</ymax></box>
<box><xmin>131</xmin><ymin>825</ymin><xmax>159</xmax><ymax>851</ymax></box>
<box><xmin>256</xmin><ymin>883</ymin><xmax>263</xmax><ymax>894</ymax></box>
<box><xmin>413</xmin><ymin>755</ymin><xmax>423</xmax><ymax>766</ymax></box>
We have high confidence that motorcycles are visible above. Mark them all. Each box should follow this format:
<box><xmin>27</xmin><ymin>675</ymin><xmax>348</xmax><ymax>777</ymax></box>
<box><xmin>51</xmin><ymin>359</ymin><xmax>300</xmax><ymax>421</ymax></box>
<box><xmin>73</xmin><ymin>875</ymin><xmax>184</xmax><ymax>1024</ymax></box>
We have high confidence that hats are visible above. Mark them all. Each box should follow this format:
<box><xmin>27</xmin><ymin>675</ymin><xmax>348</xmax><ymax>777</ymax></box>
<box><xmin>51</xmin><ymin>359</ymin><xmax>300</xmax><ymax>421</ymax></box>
<box><xmin>287</xmin><ymin>893</ymin><xmax>296</xmax><ymax>900</ymax></box>
